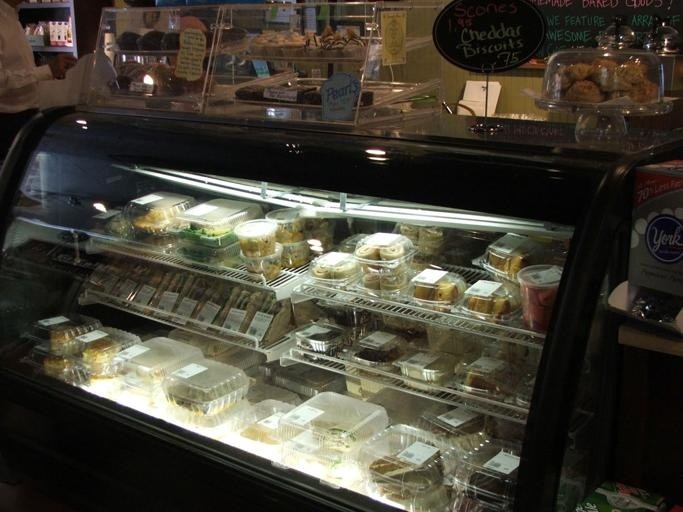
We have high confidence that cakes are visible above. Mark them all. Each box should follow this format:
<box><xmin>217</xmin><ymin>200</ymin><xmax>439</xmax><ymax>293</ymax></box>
<box><xmin>252</xmin><ymin>28</ymin><xmax>365</xmax><ymax>58</ymax></box>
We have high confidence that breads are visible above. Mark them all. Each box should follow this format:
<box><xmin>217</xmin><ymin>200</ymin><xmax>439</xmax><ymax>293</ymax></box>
<box><xmin>31</xmin><ymin>207</ymin><xmax>563</xmax><ymax>512</ymax></box>
<box><xmin>234</xmin><ymin>82</ymin><xmax>374</xmax><ymax>108</ymax></box>
<box><xmin>552</xmin><ymin>59</ymin><xmax>658</xmax><ymax>103</ymax></box>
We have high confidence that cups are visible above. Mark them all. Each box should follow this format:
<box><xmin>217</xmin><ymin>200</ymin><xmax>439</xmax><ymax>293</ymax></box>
<box><xmin>245</xmin><ymin>39</ymin><xmax>367</xmax><ymax>60</ymax></box>
<box><xmin>517</xmin><ymin>264</ymin><xmax>564</xmax><ymax>331</ymax></box>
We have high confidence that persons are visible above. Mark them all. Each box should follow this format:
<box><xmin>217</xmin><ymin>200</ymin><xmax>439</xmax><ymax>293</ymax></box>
<box><xmin>0</xmin><ymin>2</ymin><xmax>75</xmax><ymax>160</ymax></box>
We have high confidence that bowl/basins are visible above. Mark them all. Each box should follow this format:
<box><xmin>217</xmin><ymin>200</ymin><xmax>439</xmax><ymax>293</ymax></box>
<box><xmin>234</xmin><ymin>200</ymin><xmax>336</xmax><ymax>285</ymax></box>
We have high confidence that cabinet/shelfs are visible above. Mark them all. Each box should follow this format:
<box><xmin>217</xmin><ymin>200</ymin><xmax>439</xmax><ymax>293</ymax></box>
<box><xmin>11</xmin><ymin>0</ymin><xmax>105</xmax><ymax>81</ymax></box>
<box><xmin>198</xmin><ymin>0</ymin><xmax>446</xmax><ymax>129</ymax></box>
<box><xmin>0</xmin><ymin>102</ymin><xmax>682</xmax><ymax>510</ymax></box>
<box><xmin>75</xmin><ymin>3</ymin><xmax>234</xmax><ymax>117</ymax></box>
